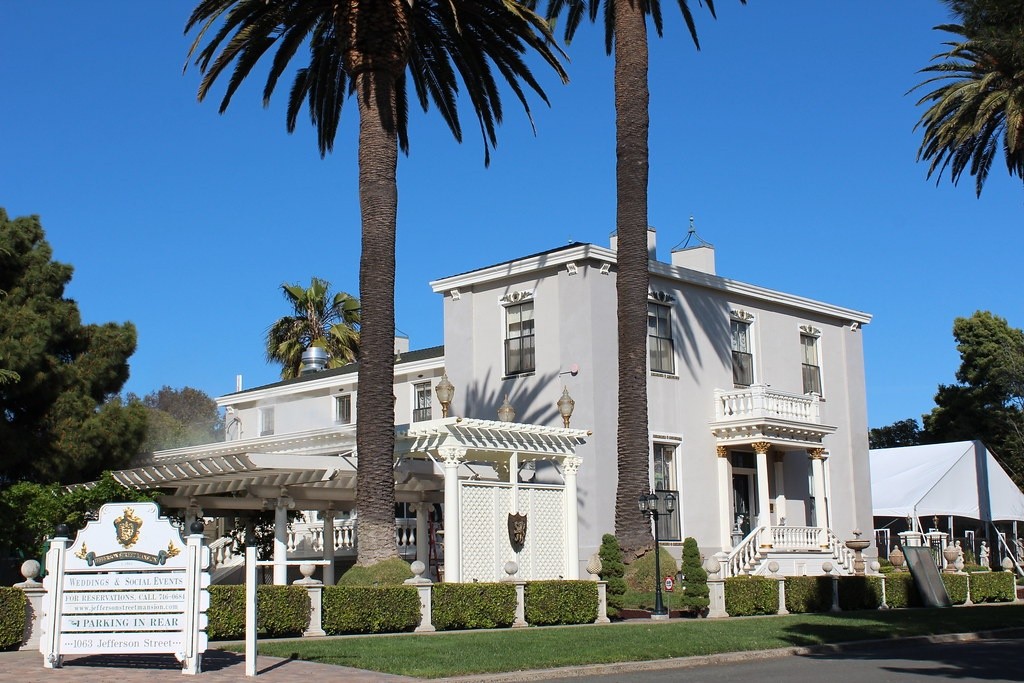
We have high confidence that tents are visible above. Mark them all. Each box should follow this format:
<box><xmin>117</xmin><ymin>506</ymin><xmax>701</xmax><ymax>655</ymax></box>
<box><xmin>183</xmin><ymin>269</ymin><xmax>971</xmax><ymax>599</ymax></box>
<box><xmin>869</xmin><ymin>439</ymin><xmax>1024</xmax><ymax>576</ymax></box>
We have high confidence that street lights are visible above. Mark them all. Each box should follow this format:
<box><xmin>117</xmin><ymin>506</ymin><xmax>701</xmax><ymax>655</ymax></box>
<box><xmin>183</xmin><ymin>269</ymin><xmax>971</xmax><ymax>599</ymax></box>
<box><xmin>497</xmin><ymin>392</ymin><xmax>516</xmax><ymax>423</ymax></box>
<box><xmin>556</xmin><ymin>385</ymin><xmax>577</xmax><ymax>428</ymax></box>
<box><xmin>639</xmin><ymin>488</ymin><xmax>677</xmax><ymax>614</ymax></box>
<box><xmin>434</xmin><ymin>371</ymin><xmax>455</xmax><ymax>420</ymax></box>
<box><xmin>932</xmin><ymin>513</ymin><xmax>940</xmax><ymax>532</ymax></box>
<box><xmin>906</xmin><ymin>513</ymin><xmax>912</xmax><ymax>531</ymax></box>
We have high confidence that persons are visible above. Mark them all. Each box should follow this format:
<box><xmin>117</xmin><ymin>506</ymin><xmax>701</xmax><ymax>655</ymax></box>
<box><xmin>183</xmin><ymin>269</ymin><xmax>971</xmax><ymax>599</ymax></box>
<box><xmin>948</xmin><ymin>540</ymin><xmax>964</xmax><ymax>563</ymax></box>
<box><xmin>980</xmin><ymin>541</ymin><xmax>989</xmax><ymax>567</ymax></box>
<box><xmin>1017</xmin><ymin>538</ymin><xmax>1024</xmax><ymax>561</ymax></box>
